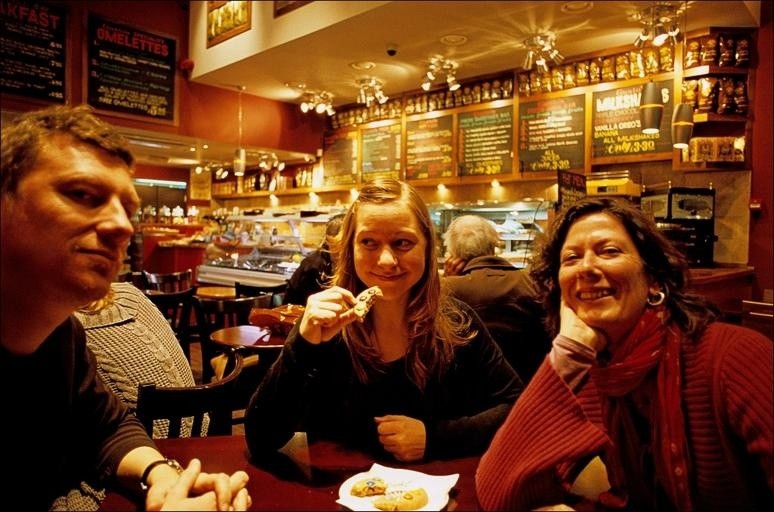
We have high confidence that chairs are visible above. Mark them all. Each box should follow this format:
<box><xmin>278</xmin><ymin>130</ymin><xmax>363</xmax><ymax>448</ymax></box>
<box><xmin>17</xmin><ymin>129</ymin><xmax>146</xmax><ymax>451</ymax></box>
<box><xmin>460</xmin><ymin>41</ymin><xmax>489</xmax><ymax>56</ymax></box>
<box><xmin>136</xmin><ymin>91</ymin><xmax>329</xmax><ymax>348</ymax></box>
<box><xmin>119</xmin><ymin>269</ymin><xmax>291</xmax><ymax>439</ymax></box>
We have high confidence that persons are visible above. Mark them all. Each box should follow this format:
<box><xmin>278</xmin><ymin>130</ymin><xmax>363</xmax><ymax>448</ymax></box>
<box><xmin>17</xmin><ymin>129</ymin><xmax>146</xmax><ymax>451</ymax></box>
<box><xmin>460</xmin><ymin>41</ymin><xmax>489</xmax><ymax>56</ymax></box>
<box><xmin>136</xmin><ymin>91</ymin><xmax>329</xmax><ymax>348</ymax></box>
<box><xmin>476</xmin><ymin>196</ymin><xmax>774</xmax><ymax>512</ymax></box>
<box><xmin>0</xmin><ymin>102</ymin><xmax>254</xmax><ymax>512</ymax></box>
<box><xmin>244</xmin><ymin>182</ymin><xmax>524</xmax><ymax>462</ymax></box>
<box><xmin>282</xmin><ymin>213</ymin><xmax>556</xmax><ymax>388</ymax></box>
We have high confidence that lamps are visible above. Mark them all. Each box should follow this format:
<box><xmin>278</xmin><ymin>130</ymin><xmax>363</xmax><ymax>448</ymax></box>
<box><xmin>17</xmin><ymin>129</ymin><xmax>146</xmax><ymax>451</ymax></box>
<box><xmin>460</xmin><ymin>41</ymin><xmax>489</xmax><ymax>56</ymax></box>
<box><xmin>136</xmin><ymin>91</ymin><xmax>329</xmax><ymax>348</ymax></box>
<box><xmin>232</xmin><ymin>85</ymin><xmax>247</xmax><ymax>177</ymax></box>
<box><xmin>420</xmin><ymin>54</ymin><xmax>460</xmax><ymax>93</ymax></box>
<box><xmin>520</xmin><ymin>34</ymin><xmax>564</xmax><ymax>75</ymax></box>
<box><xmin>632</xmin><ymin>6</ymin><xmax>695</xmax><ymax>150</ymax></box>
<box><xmin>351</xmin><ymin>76</ymin><xmax>389</xmax><ymax>109</ymax></box>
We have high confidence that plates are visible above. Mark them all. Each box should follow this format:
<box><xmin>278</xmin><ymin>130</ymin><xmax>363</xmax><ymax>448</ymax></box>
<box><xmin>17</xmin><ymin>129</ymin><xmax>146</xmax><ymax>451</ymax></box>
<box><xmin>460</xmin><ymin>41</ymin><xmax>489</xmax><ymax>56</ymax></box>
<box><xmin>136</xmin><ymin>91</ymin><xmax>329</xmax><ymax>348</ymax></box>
<box><xmin>337</xmin><ymin>471</ymin><xmax>449</xmax><ymax>511</ymax></box>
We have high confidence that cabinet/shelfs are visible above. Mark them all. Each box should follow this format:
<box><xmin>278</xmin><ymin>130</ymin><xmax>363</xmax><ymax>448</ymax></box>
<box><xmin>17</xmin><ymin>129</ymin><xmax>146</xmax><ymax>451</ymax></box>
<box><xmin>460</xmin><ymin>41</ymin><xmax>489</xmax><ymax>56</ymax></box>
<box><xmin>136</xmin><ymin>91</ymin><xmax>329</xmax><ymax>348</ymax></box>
<box><xmin>194</xmin><ymin>200</ymin><xmax>548</xmax><ymax>286</ymax></box>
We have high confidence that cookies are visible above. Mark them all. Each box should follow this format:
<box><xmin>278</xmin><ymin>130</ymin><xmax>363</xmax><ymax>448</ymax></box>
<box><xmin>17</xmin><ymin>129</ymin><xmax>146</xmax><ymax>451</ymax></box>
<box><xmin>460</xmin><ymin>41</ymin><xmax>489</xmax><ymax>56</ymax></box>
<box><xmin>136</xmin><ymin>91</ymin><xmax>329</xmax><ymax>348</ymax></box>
<box><xmin>352</xmin><ymin>285</ymin><xmax>384</xmax><ymax>323</ymax></box>
<box><xmin>352</xmin><ymin>478</ymin><xmax>428</xmax><ymax>512</ymax></box>
